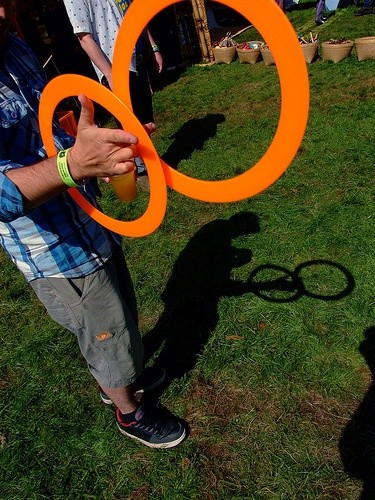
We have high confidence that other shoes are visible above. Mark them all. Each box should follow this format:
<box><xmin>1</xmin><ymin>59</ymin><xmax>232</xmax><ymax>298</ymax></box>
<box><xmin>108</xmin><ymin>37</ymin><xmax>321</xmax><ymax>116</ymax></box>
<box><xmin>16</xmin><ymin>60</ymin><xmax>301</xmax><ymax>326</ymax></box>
<box><xmin>354</xmin><ymin>7</ymin><xmax>372</xmax><ymax>16</ymax></box>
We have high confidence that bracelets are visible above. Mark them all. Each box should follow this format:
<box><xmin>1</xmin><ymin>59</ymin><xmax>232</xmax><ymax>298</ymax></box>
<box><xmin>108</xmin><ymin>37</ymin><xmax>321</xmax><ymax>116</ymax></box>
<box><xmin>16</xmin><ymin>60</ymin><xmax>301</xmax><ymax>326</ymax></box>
<box><xmin>57</xmin><ymin>148</ymin><xmax>88</xmax><ymax>187</ymax></box>
<box><xmin>152</xmin><ymin>45</ymin><xmax>159</xmax><ymax>52</ymax></box>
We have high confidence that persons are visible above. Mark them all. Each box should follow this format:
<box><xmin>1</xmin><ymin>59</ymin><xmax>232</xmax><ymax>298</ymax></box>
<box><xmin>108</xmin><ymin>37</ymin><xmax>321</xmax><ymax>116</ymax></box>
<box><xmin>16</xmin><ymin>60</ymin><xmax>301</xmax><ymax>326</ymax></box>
<box><xmin>63</xmin><ymin>0</ymin><xmax>164</xmax><ymax>192</ymax></box>
<box><xmin>353</xmin><ymin>0</ymin><xmax>375</xmax><ymax>16</ymax></box>
<box><xmin>0</xmin><ymin>0</ymin><xmax>186</xmax><ymax>449</ymax></box>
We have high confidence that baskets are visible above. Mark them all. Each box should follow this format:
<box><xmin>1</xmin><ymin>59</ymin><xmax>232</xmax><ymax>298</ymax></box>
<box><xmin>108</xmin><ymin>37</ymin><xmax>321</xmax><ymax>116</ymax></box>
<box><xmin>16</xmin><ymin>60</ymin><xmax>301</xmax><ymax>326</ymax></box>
<box><xmin>301</xmin><ymin>43</ymin><xmax>318</xmax><ymax>63</ymax></box>
<box><xmin>214</xmin><ymin>40</ymin><xmax>238</xmax><ymax>65</ymax></box>
<box><xmin>354</xmin><ymin>37</ymin><xmax>375</xmax><ymax>61</ymax></box>
<box><xmin>260</xmin><ymin>45</ymin><xmax>275</xmax><ymax>66</ymax></box>
<box><xmin>321</xmin><ymin>40</ymin><xmax>354</xmax><ymax>64</ymax></box>
<box><xmin>235</xmin><ymin>41</ymin><xmax>264</xmax><ymax>64</ymax></box>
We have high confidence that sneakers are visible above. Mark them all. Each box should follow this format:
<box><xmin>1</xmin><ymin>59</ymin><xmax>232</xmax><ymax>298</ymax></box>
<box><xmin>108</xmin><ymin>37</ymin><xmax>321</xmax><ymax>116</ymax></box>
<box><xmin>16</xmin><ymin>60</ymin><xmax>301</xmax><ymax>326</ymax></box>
<box><xmin>100</xmin><ymin>365</ymin><xmax>165</xmax><ymax>403</ymax></box>
<box><xmin>116</xmin><ymin>398</ymin><xmax>186</xmax><ymax>449</ymax></box>
